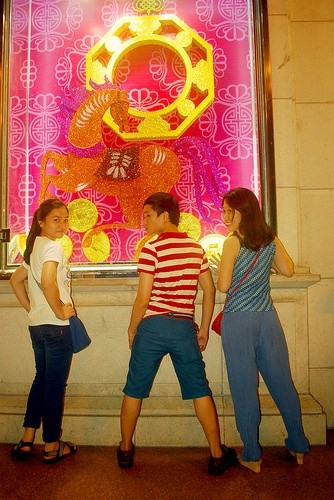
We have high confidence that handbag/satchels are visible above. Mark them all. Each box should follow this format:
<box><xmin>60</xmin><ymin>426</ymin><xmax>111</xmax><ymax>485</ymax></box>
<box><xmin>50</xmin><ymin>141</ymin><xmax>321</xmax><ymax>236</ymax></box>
<box><xmin>68</xmin><ymin>315</ymin><xmax>92</xmax><ymax>354</ymax></box>
<box><xmin>211</xmin><ymin>310</ymin><xmax>223</xmax><ymax>336</ymax></box>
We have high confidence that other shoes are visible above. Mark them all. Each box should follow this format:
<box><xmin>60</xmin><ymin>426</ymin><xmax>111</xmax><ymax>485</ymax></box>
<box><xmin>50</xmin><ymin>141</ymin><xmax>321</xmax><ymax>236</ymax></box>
<box><xmin>207</xmin><ymin>443</ymin><xmax>237</xmax><ymax>476</ymax></box>
<box><xmin>116</xmin><ymin>440</ymin><xmax>136</xmax><ymax>468</ymax></box>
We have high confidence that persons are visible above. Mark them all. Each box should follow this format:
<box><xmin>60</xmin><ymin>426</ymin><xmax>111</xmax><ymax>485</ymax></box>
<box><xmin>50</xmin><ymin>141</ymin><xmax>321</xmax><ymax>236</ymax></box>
<box><xmin>216</xmin><ymin>186</ymin><xmax>312</xmax><ymax>476</ymax></box>
<box><xmin>8</xmin><ymin>197</ymin><xmax>78</xmax><ymax>464</ymax></box>
<box><xmin>114</xmin><ymin>190</ymin><xmax>238</xmax><ymax>477</ymax></box>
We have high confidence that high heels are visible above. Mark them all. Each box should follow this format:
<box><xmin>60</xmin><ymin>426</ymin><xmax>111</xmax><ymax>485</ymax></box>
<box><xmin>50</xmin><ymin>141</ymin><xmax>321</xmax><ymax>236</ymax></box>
<box><xmin>239</xmin><ymin>459</ymin><xmax>262</xmax><ymax>473</ymax></box>
<box><xmin>289</xmin><ymin>449</ymin><xmax>304</xmax><ymax>465</ymax></box>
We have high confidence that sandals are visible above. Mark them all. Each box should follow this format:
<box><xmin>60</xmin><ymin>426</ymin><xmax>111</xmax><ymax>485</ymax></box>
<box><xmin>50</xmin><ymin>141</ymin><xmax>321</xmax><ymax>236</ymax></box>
<box><xmin>11</xmin><ymin>439</ymin><xmax>34</xmax><ymax>460</ymax></box>
<box><xmin>42</xmin><ymin>440</ymin><xmax>77</xmax><ymax>465</ymax></box>
